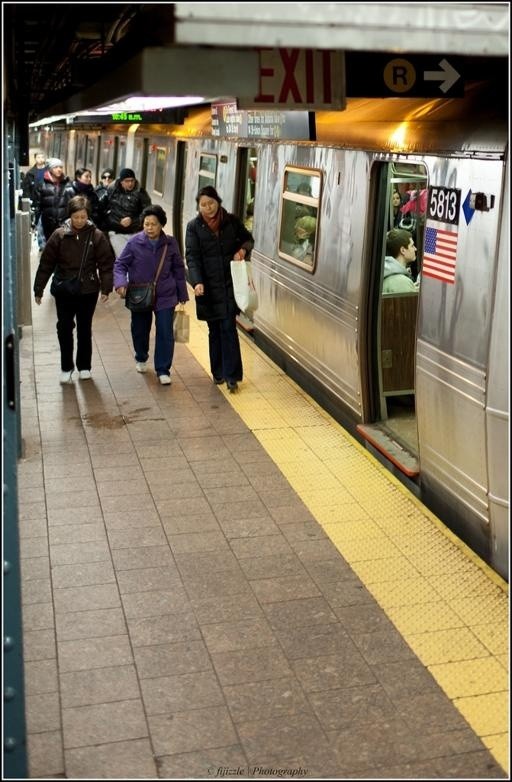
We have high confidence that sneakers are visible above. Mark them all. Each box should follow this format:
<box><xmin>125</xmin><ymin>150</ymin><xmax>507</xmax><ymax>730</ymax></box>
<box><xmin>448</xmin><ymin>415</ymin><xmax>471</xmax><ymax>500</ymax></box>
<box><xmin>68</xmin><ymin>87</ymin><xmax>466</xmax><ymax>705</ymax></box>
<box><xmin>59</xmin><ymin>365</ymin><xmax>75</xmax><ymax>386</ymax></box>
<box><xmin>212</xmin><ymin>366</ymin><xmax>226</xmax><ymax>388</ymax></box>
<box><xmin>158</xmin><ymin>367</ymin><xmax>172</xmax><ymax>386</ymax></box>
<box><xmin>135</xmin><ymin>353</ymin><xmax>149</xmax><ymax>376</ymax></box>
<box><xmin>226</xmin><ymin>375</ymin><xmax>240</xmax><ymax>391</ymax></box>
<box><xmin>78</xmin><ymin>365</ymin><xmax>91</xmax><ymax>381</ymax></box>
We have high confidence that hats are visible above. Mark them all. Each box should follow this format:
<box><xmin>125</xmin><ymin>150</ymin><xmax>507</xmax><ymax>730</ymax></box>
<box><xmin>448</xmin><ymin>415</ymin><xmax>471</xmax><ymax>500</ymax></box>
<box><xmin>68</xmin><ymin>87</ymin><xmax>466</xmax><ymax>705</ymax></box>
<box><xmin>119</xmin><ymin>168</ymin><xmax>136</xmax><ymax>182</ymax></box>
<box><xmin>46</xmin><ymin>157</ymin><xmax>64</xmax><ymax>170</ymax></box>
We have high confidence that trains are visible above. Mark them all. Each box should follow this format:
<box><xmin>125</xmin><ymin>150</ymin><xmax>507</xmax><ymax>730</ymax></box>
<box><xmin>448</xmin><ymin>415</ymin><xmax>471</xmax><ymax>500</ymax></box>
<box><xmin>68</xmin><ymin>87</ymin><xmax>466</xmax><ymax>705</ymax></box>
<box><xmin>27</xmin><ymin>88</ymin><xmax>511</xmax><ymax>584</ymax></box>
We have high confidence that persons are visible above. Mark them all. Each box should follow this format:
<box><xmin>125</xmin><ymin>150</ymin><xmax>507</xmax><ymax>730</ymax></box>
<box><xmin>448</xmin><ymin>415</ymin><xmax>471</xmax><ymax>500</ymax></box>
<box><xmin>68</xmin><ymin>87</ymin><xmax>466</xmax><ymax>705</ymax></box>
<box><xmin>185</xmin><ymin>185</ymin><xmax>255</xmax><ymax>392</ymax></box>
<box><xmin>383</xmin><ymin>189</ymin><xmax>427</xmax><ymax>292</ymax></box>
<box><xmin>113</xmin><ymin>205</ymin><xmax>189</xmax><ymax>384</ymax></box>
<box><xmin>34</xmin><ymin>196</ymin><xmax>116</xmax><ymax>385</ymax></box>
<box><xmin>247</xmin><ymin>159</ymin><xmax>316</xmax><ymax>266</ymax></box>
<box><xmin>23</xmin><ymin>153</ymin><xmax>151</xmax><ymax>259</ymax></box>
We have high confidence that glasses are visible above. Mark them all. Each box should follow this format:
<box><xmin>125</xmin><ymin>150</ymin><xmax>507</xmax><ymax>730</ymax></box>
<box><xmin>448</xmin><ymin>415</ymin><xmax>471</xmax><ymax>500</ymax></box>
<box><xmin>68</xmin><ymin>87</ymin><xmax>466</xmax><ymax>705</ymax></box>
<box><xmin>101</xmin><ymin>175</ymin><xmax>114</xmax><ymax>180</ymax></box>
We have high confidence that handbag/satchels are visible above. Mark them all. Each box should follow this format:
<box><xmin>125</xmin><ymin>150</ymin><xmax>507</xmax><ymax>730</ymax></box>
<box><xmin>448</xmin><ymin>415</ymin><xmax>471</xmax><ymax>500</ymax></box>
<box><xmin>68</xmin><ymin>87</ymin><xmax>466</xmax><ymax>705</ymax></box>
<box><xmin>123</xmin><ymin>282</ymin><xmax>156</xmax><ymax>312</ymax></box>
<box><xmin>229</xmin><ymin>259</ymin><xmax>260</xmax><ymax>313</ymax></box>
<box><xmin>50</xmin><ymin>275</ymin><xmax>82</xmax><ymax>299</ymax></box>
<box><xmin>172</xmin><ymin>309</ymin><xmax>191</xmax><ymax>344</ymax></box>
